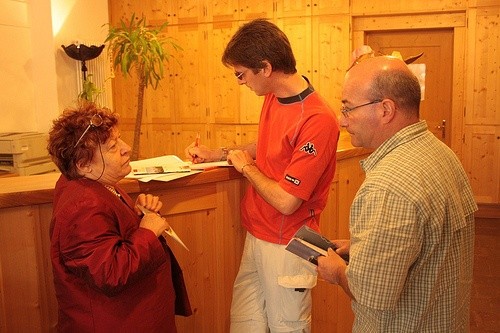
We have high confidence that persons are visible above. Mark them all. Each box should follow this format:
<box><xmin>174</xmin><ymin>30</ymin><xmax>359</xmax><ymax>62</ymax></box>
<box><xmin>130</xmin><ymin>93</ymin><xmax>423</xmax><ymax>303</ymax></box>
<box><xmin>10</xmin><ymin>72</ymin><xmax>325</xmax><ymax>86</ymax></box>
<box><xmin>44</xmin><ymin>99</ymin><xmax>193</xmax><ymax>333</ymax></box>
<box><xmin>184</xmin><ymin>20</ymin><xmax>340</xmax><ymax>333</ymax></box>
<box><xmin>317</xmin><ymin>56</ymin><xmax>479</xmax><ymax>333</ymax></box>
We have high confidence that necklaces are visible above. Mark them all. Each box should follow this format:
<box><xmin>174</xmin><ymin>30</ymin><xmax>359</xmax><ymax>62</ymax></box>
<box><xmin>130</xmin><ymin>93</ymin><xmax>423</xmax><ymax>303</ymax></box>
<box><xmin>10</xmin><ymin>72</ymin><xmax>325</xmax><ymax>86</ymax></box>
<box><xmin>105</xmin><ymin>184</ymin><xmax>121</xmax><ymax>197</ymax></box>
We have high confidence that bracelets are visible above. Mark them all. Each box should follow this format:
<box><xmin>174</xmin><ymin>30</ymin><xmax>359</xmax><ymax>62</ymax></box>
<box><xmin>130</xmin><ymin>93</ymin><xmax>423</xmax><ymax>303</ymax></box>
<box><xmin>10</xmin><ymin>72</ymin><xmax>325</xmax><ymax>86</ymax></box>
<box><xmin>221</xmin><ymin>147</ymin><xmax>228</xmax><ymax>160</ymax></box>
<box><xmin>241</xmin><ymin>164</ymin><xmax>256</xmax><ymax>177</ymax></box>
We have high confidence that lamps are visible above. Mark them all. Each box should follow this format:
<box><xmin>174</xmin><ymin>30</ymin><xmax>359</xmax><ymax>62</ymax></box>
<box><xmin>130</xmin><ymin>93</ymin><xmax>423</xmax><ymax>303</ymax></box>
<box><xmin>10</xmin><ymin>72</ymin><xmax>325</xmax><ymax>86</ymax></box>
<box><xmin>62</xmin><ymin>43</ymin><xmax>105</xmax><ymax>103</ymax></box>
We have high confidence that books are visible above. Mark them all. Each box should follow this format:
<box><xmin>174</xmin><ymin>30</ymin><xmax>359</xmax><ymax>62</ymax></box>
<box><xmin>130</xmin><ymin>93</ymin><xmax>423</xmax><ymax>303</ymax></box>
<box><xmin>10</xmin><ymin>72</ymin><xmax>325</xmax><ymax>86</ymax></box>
<box><xmin>286</xmin><ymin>224</ymin><xmax>349</xmax><ymax>269</ymax></box>
<box><xmin>133</xmin><ymin>164</ymin><xmax>190</xmax><ymax>175</ymax></box>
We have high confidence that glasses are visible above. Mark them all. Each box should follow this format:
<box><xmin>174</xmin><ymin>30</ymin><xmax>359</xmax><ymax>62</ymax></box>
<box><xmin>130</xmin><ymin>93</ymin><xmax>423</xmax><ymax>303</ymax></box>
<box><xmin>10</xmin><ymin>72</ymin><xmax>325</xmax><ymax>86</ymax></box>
<box><xmin>340</xmin><ymin>99</ymin><xmax>383</xmax><ymax>118</ymax></box>
<box><xmin>72</xmin><ymin>106</ymin><xmax>111</xmax><ymax>148</ymax></box>
<box><xmin>235</xmin><ymin>66</ymin><xmax>256</xmax><ymax>81</ymax></box>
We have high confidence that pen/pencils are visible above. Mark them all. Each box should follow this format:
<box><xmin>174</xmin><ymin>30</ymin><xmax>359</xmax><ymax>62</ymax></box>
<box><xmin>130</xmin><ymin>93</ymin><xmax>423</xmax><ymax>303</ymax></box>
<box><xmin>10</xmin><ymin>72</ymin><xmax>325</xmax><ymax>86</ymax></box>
<box><xmin>192</xmin><ymin>138</ymin><xmax>198</xmax><ymax>165</ymax></box>
